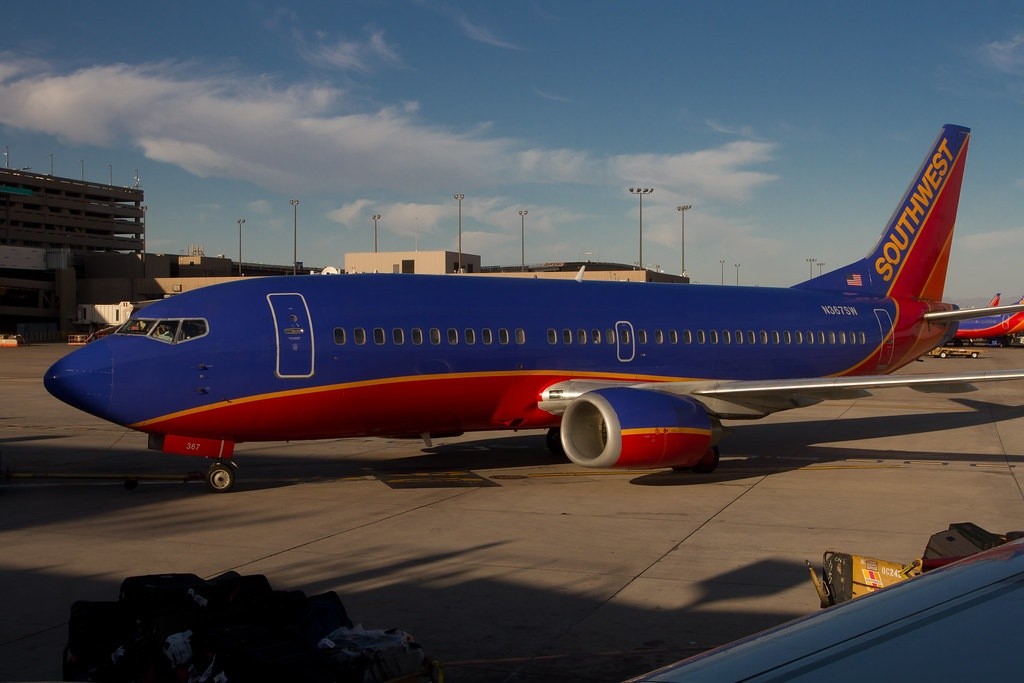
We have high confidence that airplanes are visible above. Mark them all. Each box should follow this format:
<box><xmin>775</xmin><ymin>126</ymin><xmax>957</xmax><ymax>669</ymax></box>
<box><xmin>42</xmin><ymin>123</ymin><xmax>1022</xmax><ymax>495</ymax></box>
<box><xmin>943</xmin><ymin>294</ymin><xmax>1024</xmax><ymax>347</ymax></box>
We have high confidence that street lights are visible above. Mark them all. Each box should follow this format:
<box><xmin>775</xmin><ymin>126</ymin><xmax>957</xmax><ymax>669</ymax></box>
<box><xmin>237</xmin><ymin>217</ymin><xmax>246</xmax><ymax>276</ymax></box>
<box><xmin>452</xmin><ymin>194</ymin><xmax>464</xmax><ymax>272</ymax></box>
<box><xmin>627</xmin><ymin>188</ymin><xmax>655</xmax><ymax>270</ymax></box>
<box><xmin>817</xmin><ymin>263</ymin><xmax>826</xmax><ymax>277</ymax></box>
<box><xmin>288</xmin><ymin>199</ymin><xmax>299</xmax><ymax>274</ymax></box>
<box><xmin>735</xmin><ymin>264</ymin><xmax>741</xmax><ymax>286</ymax></box>
<box><xmin>720</xmin><ymin>260</ymin><xmax>726</xmax><ymax>286</ymax></box>
<box><xmin>372</xmin><ymin>214</ymin><xmax>382</xmax><ymax>253</ymax></box>
<box><xmin>806</xmin><ymin>258</ymin><xmax>818</xmax><ymax>278</ymax></box>
<box><xmin>518</xmin><ymin>210</ymin><xmax>529</xmax><ymax>272</ymax></box>
<box><xmin>675</xmin><ymin>204</ymin><xmax>692</xmax><ymax>278</ymax></box>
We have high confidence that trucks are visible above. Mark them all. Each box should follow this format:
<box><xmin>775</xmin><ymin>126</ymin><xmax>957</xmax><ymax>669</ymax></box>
<box><xmin>926</xmin><ymin>347</ymin><xmax>988</xmax><ymax>358</ymax></box>
